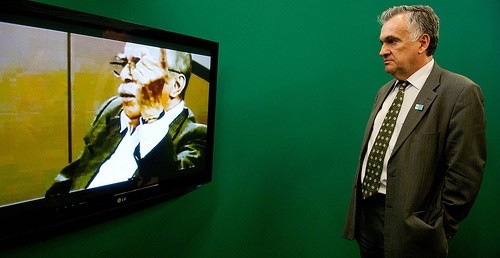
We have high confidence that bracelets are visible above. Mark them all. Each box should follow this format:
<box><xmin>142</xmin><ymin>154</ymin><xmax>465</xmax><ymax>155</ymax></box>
<box><xmin>141</xmin><ymin>109</ymin><xmax>165</xmax><ymax>124</ymax></box>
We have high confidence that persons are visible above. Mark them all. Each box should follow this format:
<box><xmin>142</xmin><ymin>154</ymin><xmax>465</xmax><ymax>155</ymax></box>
<box><xmin>342</xmin><ymin>3</ymin><xmax>488</xmax><ymax>258</ymax></box>
<box><xmin>43</xmin><ymin>41</ymin><xmax>208</xmax><ymax>200</ymax></box>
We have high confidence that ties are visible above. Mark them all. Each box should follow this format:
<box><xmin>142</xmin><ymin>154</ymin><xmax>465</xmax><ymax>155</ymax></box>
<box><xmin>360</xmin><ymin>80</ymin><xmax>411</xmax><ymax>200</ymax></box>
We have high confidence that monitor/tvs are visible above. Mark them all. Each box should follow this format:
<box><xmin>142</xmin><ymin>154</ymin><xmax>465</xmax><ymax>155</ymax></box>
<box><xmin>0</xmin><ymin>0</ymin><xmax>220</xmax><ymax>249</ymax></box>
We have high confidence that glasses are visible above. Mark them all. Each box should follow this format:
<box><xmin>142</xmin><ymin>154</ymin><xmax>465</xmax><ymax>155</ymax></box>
<box><xmin>110</xmin><ymin>54</ymin><xmax>188</xmax><ymax>79</ymax></box>
